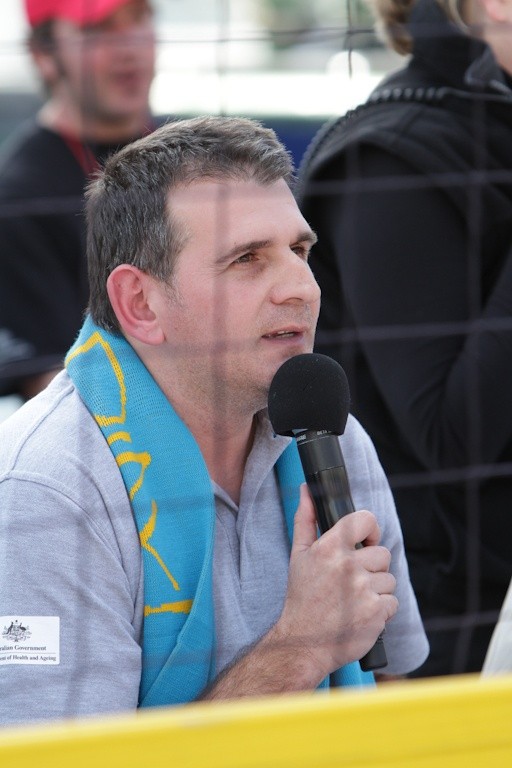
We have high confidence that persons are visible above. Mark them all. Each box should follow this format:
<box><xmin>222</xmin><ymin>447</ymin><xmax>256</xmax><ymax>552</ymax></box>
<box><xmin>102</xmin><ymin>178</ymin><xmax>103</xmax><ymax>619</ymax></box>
<box><xmin>289</xmin><ymin>0</ymin><xmax>512</xmax><ymax>681</ymax></box>
<box><xmin>0</xmin><ymin>0</ymin><xmax>162</xmax><ymax>406</ymax></box>
<box><xmin>0</xmin><ymin>113</ymin><xmax>431</xmax><ymax>729</ymax></box>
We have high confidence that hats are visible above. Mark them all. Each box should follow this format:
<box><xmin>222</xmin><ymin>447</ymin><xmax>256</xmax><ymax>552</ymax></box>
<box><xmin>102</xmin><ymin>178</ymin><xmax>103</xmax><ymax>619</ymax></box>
<box><xmin>25</xmin><ymin>0</ymin><xmax>128</xmax><ymax>25</ymax></box>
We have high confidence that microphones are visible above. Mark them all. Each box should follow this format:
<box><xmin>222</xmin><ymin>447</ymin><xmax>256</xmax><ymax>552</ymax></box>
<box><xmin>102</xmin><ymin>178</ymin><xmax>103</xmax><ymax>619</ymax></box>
<box><xmin>268</xmin><ymin>353</ymin><xmax>384</xmax><ymax>641</ymax></box>
